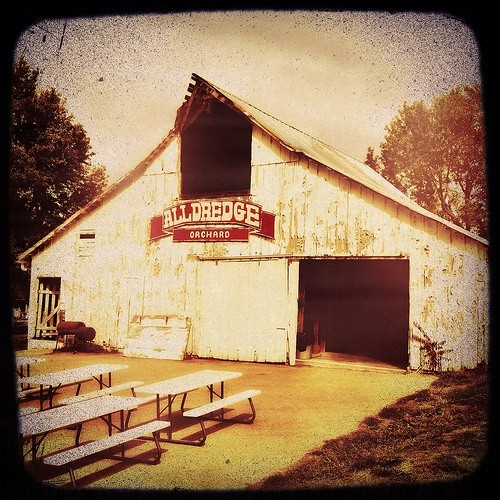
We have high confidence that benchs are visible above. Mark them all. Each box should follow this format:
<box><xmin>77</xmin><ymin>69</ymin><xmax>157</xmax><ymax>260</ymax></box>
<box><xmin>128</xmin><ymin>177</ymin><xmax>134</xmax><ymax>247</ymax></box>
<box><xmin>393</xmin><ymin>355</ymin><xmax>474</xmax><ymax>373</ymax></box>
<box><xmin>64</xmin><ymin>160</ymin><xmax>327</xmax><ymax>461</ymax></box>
<box><xmin>58</xmin><ymin>380</ymin><xmax>144</xmax><ymax>406</ymax></box>
<box><xmin>183</xmin><ymin>389</ymin><xmax>262</xmax><ymax>446</ymax></box>
<box><xmin>22</xmin><ymin>377</ymin><xmax>93</xmax><ymax>408</ymax></box>
<box><xmin>42</xmin><ymin>419</ymin><xmax>170</xmax><ymax>489</ymax></box>
<box><xmin>19</xmin><ymin>406</ymin><xmax>39</xmax><ymax>417</ymax></box>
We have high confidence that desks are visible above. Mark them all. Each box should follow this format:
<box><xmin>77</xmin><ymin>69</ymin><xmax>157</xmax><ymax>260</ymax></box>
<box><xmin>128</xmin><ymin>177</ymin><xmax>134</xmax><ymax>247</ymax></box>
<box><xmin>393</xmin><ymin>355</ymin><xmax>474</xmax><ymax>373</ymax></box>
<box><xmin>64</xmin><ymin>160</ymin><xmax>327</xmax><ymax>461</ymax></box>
<box><xmin>15</xmin><ymin>356</ymin><xmax>46</xmax><ymax>378</ymax></box>
<box><xmin>20</xmin><ymin>394</ymin><xmax>144</xmax><ymax>467</ymax></box>
<box><xmin>19</xmin><ymin>362</ymin><xmax>129</xmax><ymax>410</ymax></box>
<box><xmin>134</xmin><ymin>369</ymin><xmax>243</xmax><ymax>445</ymax></box>
